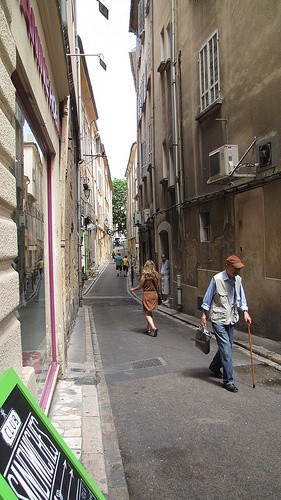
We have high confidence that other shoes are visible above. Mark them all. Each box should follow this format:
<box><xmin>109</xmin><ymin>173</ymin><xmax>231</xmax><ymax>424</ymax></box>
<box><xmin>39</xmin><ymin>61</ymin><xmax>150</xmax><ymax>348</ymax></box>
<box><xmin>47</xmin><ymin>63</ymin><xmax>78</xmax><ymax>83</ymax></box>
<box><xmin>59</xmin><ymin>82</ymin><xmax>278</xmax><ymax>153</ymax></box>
<box><xmin>126</xmin><ymin>276</ymin><xmax>128</xmax><ymax>277</ymax></box>
<box><xmin>144</xmin><ymin>330</ymin><xmax>151</xmax><ymax>335</ymax></box>
<box><xmin>124</xmin><ymin>275</ymin><xmax>125</xmax><ymax>277</ymax></box>
<box><xmin>153</xmin><ymin>327</ymin><xmax>158</xmax><ymax>337</ymax></box>
<box><xmin>120</xmin><ymin>275</ymin><xmax>122</xmax><ymax>277</ymax></box>
<box><xmin>164</xmin><ymin>299</ymin><xmax>169</xmax><ymax>304</ymax></box>
<box><xmin>117</xmin><ymin>273</ymin><xmax>119</xmax><ymax>277</ymax></box>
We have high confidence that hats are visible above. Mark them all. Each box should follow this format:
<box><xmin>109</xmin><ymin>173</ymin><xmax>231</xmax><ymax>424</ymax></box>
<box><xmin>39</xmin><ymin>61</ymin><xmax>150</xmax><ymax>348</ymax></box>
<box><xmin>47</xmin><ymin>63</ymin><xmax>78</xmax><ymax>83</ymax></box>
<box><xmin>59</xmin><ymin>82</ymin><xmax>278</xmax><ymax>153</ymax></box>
<box><xmin>225</xmin><ymin>255</ymin><xmax>245</xmax><ymax>269</ymax></box>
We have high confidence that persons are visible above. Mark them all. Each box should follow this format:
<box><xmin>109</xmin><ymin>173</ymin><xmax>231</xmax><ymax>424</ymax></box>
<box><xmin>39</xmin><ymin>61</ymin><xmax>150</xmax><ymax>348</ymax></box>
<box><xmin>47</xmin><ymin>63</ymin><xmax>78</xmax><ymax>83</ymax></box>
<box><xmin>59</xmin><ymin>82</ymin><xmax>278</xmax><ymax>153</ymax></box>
<box><xmin>160</xmin><ymin>254</ymin><xmax>170</xmax><ymax>303</ymax></box>
<box><xmin>200</xmin><ymin>255</ymin><xmax>252</xmax><ymax>393</ymax></box>
<box><xmin>129</xmin><ymin>260</ymin><xmax>160</xmax><ymax>337</ymax></box>
<box><xmin>112</xmin><ymin>250</ymin><xmax>130</xmax><ymax>277</ymax></box>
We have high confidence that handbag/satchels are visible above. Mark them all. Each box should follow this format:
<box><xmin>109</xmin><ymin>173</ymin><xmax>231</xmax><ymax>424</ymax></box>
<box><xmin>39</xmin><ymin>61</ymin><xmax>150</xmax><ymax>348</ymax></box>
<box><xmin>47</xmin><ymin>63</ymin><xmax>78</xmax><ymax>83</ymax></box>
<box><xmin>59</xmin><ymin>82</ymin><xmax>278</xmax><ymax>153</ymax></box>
<box><xmin>158</xmin><ymin>293</ymin><xmax>162</xmax><ymax>305</ymax></box>
<box><xmin>195</xmin><ymin>324</ymin><xmax>211</xmax><ymax>355</ymax></box>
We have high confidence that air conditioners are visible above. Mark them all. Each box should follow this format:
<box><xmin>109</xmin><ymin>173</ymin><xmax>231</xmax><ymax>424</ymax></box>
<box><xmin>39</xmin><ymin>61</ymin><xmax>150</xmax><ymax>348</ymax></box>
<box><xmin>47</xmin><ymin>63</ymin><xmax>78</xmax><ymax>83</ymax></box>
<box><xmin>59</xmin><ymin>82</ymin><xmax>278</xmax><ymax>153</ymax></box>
<box><xmin>208</xmin><ymin>144</ymin><xmax>239</xmax><ymax>181</ymax></box>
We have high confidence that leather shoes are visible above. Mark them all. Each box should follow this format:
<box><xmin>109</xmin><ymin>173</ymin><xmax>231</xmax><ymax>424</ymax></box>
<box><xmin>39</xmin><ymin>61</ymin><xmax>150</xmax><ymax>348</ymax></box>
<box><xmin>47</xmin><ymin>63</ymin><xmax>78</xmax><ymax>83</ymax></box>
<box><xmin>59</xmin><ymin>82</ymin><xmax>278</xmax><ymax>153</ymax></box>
<box><xmin>223</xmin><ymin>382</ymin><xmax>238</xmax><ymax>393</ymax></box>
<box><xmin>209</xmin><ymin>364</ymin><xmax>223</xmax><ymax>378</ymax></box>
